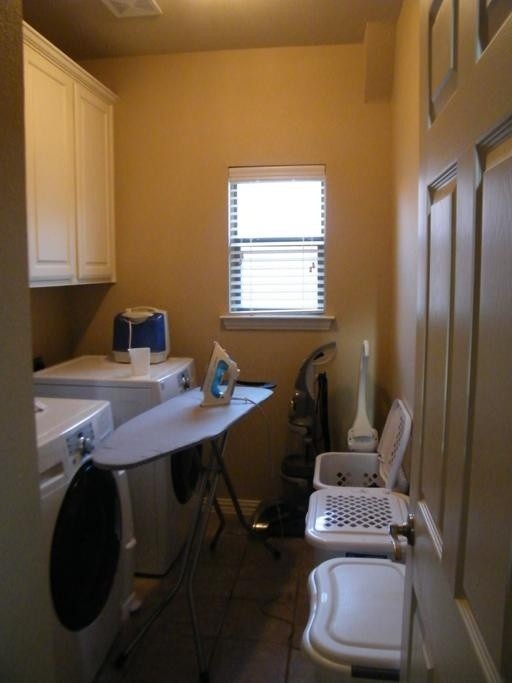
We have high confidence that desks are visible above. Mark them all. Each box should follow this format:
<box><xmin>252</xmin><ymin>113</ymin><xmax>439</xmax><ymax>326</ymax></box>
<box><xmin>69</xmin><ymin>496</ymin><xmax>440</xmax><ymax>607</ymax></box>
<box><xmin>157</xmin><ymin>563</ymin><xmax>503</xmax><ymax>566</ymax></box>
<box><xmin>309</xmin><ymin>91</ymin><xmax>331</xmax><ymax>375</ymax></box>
<box><xmin>92</xmin><ymin>384</ymin><xmax>274</xmax><ymax>681</ymax></box>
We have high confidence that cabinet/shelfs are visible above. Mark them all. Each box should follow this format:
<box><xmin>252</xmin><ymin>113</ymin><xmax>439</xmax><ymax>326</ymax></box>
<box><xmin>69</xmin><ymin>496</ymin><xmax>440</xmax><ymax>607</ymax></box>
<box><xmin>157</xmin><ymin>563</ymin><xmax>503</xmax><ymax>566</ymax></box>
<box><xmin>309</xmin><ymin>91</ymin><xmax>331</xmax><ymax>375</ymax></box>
<box><xmin>22</xmin><ymin>19</ymin><xmax>116</xmax><ymax>288</ymax></box>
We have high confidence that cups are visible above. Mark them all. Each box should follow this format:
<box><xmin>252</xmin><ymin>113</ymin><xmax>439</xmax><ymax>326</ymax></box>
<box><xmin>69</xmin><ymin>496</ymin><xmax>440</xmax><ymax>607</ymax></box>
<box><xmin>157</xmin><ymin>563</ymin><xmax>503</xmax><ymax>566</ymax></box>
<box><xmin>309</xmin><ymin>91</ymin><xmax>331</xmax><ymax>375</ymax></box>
<box><xmin>128</xmin><ymin>346</ymin><xmax>151</xmax><ymax>376</ymax></box>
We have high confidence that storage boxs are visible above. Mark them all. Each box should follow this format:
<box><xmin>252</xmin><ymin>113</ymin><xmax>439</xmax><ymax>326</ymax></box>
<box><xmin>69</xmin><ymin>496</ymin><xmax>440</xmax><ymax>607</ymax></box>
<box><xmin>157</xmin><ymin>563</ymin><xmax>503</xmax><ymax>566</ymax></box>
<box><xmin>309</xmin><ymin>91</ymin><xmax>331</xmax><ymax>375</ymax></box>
<box><xmin>301</xmin><ymin>397</ymin><xmax>412</xmax><ymax>679</ymax></box>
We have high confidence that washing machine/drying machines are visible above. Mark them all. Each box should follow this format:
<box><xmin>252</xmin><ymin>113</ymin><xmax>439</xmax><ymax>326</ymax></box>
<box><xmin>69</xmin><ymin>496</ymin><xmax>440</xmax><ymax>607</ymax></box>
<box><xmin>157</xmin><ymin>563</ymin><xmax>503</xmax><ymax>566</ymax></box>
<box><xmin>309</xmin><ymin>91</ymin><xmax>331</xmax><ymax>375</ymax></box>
<box><xmin>32</xmin><ymin>396</ymin><xmax>134</xmax><ymax>682</ymax></box>
<box><xmin>33</xmin><ymin>354</ymin><xmax>198</xmax><ymax>575</ymax></box>
